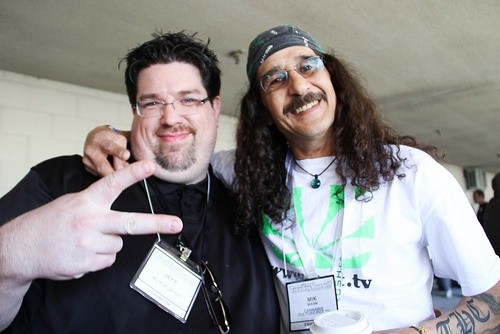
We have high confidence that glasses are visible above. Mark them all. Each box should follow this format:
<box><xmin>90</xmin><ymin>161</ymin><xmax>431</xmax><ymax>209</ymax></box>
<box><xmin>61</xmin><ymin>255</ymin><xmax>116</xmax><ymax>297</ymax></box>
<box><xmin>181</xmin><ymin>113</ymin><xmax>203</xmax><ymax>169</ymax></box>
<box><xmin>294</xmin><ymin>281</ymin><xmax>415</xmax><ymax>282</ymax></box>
<box><xmin>200</xmin><ymin>260</ymin><xmax>229</xmax><ymax>334</ymax></box>
<box><xmin>256</xmin><ymin>56</ymin><xmax>324</xmax><ymax>93</ymax></box>
<box><xmin>133</xmin><ymin>94</ymin><xmax>211</xmax><ymax>118</ymax></box>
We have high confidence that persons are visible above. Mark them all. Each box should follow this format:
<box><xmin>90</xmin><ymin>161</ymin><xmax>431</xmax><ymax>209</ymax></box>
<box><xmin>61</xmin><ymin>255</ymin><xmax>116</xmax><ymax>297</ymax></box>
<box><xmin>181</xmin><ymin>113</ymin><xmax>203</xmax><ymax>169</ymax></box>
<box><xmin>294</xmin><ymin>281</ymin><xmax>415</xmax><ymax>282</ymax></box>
<box><xmin>1</xmin><ymin>29</ymin><xmax>281</xmax><ymax>334</ymax></box>
<box><xmin>470</xmin><ymin>173</ymin><xmax>500</xmax><ymax>258</ymax></box>
<box><xmin>473</xmin><ymin>189</ymin><xmax>489</xmax><ymax>225</ymax></box>
<box><xmin>82</xmin><ymin>25</ymin><xmax>500</xmax><ymax>334</ymax></box>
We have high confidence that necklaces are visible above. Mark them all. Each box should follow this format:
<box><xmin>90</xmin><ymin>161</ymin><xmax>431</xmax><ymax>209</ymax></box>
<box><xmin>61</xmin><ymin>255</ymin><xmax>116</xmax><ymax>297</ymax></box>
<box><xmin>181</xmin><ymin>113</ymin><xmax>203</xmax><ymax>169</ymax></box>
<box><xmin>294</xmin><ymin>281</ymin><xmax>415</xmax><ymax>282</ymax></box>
<box><xmin>286</xmin><ymin>142</ymin><xmax>342</xmax><ymax>191</ymax></box>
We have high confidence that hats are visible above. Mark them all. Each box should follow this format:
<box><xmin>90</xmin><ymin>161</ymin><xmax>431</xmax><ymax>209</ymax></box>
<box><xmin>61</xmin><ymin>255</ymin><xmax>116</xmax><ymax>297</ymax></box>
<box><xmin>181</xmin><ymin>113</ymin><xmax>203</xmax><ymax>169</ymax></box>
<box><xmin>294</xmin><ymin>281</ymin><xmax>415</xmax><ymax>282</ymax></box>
<box><xmin>247</xmin><ymin>26</ymin><xmax>325</xmax><ymax>87</ymax></box>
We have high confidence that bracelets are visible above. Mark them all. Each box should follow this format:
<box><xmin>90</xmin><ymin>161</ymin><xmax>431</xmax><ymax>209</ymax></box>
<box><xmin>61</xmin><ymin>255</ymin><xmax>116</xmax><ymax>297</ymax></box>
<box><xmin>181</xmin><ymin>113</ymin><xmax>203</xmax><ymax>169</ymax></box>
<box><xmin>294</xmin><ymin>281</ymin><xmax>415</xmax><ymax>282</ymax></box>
<box><xmin>104</xmin><ymin>125</ymin><xmax>120</xmax><ymax>134</ymax></box>
<box><xmin>409</xmin><ymin>323</ymin><xmax>430</xmax><ymax>334</ymax></box>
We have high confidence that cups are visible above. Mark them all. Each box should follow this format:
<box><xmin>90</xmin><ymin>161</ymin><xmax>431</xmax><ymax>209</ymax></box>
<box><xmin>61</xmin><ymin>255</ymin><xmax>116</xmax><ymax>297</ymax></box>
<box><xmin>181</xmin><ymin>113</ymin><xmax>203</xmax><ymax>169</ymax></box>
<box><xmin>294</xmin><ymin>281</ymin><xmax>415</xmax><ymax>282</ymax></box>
<box><xmin>309</xmin><ymin>309</ymin><xmax>373</xmax><ymax>334</ymax></box>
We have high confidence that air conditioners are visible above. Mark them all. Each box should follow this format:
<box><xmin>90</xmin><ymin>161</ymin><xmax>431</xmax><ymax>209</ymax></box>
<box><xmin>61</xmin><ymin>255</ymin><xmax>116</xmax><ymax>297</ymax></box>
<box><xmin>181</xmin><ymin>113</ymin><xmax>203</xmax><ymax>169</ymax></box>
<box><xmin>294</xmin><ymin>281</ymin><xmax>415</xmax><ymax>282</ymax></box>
<box><xmin>464</xmin><ymin>165</ymin><xmax>488</xmax><ymax>192</ymax></box>
